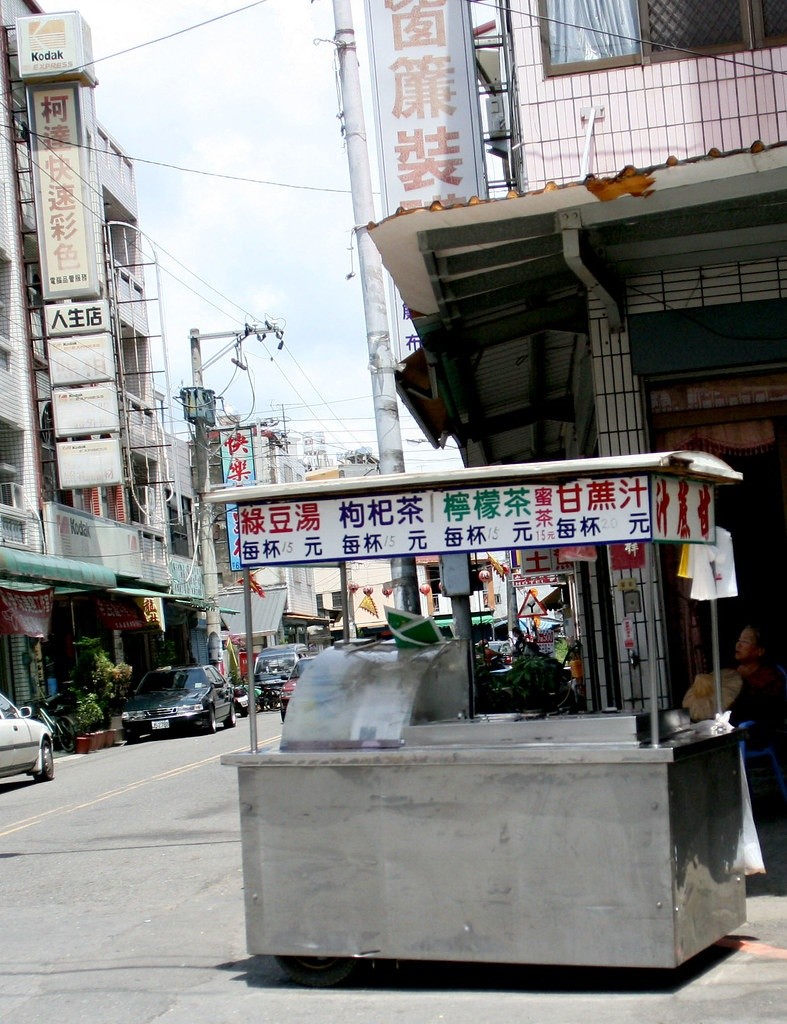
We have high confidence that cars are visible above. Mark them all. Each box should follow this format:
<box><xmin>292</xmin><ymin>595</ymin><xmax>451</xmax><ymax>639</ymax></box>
<box><xmin>119</xmin><ymin>663</ymin><xmax>236</xmax><ymax>743</ymax></box>
<box><xmin>475</xmin><ymin>641</ymin><xmax>513</xmax><ymax>669</ymax></box>
<box><xmin>0</xmin><ymin>694</ymin><xmax>54</xmax><ymax>782</ymax></box>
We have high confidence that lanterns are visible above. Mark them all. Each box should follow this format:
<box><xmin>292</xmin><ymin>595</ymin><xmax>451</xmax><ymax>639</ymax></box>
<box><xmin>348</xmin><ymin>560</ymin><xmax>513</xmax><ymax>603</ymax></box>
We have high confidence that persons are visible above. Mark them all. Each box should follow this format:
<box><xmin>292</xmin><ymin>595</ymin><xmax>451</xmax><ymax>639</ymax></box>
<box><xmin>726</xmin><ymin>622</ymin><xmax>786</xmax><ymax>752</ymax></box>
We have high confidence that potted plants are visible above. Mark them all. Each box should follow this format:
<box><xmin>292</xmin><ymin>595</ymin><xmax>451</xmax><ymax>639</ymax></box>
<box><xmin>63</xmin><ymin>635</ymin><xmax>133</xmax><ymax>754</ymax></box>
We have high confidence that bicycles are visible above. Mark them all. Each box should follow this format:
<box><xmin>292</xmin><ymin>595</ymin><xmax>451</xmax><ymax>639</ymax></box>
<box><xmin>238</xmin><ymin>673</ymin><xmax>283</xmax><ymax>712</ymax></box>
<box><xmin>23</xmin><ymin>695</ymin><xmax>77</xmax><ymax>754</ymax></box>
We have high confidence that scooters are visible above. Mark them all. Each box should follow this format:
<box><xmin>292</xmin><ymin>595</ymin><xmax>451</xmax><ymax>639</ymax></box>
<box><xmin>225</xmin><ymin>671</ymin><xmax>249</xmax><ymax>718</ymax></box>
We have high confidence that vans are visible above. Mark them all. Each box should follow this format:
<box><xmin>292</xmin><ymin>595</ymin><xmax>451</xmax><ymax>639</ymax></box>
<box><xmin>254</xmin><ymin>643</ymin><xmax>309</xmax><ymax>680</ymax></box>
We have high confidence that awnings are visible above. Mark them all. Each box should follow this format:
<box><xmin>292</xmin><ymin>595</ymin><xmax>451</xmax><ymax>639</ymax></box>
<box><xmin>217</xmin><ymin>581</ymin><xmax>344</xmax><ymax>643</ymax></box>
<box><xmin>97</xmin><ymin>578</ymin><xmax>242</xmax><ymax>634</ymax></box>
<box><xmin>411</xmin><ymin>270</ymin><xmax>588</xmax><ymax>477</ymax></box>
<box><xmin>433</xmin><ymin>615</ymin><xmax>563</xmax><ymax>634</ymax></box>
<box><xmin>0</xmin><ymin>546</ymin><xmax>118</xmax><ymax>597</ymax></box>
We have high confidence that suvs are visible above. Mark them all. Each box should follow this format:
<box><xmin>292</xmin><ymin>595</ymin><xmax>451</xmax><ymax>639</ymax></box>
<box><xmin>279</xmin><ymin>656</ymin><xmax>317</xmax><ymax>722</ymax></box>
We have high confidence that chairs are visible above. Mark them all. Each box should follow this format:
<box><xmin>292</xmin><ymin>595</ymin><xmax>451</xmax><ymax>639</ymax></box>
<box><xmin>736</xmin><ymin>664</ymin><xmax>787</xmax><ymax>801</ymax></box>
